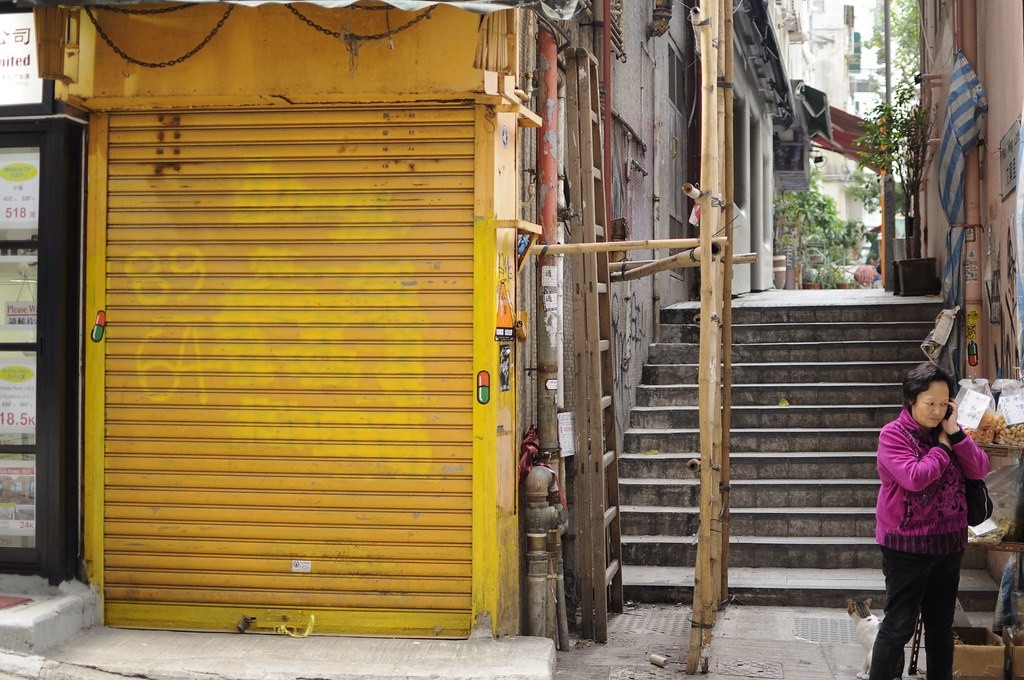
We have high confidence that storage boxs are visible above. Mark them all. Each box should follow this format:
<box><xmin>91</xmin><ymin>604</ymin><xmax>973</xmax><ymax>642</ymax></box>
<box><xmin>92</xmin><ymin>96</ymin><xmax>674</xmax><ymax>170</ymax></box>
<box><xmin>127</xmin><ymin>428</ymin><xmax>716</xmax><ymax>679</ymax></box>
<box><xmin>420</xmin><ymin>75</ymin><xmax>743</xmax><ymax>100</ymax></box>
<box><xmin>950</xmin><ymin>626</ymin><xmax>1005</xmax><ymax>680</ymax></box>
<box><xmin>1002</xmin><ymin>625</ymin><xmax>1024</xmax><ymax>680</ymax></box>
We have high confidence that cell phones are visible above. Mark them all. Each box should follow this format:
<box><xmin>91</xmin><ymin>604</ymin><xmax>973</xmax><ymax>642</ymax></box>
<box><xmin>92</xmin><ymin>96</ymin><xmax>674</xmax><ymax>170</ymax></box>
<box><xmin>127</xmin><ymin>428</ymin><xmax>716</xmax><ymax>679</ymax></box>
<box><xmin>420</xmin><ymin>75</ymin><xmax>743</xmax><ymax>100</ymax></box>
<box><xmin>944</xmin><ymin>406</ymin><xmax>953</xmax><ymax>420</ymax></box>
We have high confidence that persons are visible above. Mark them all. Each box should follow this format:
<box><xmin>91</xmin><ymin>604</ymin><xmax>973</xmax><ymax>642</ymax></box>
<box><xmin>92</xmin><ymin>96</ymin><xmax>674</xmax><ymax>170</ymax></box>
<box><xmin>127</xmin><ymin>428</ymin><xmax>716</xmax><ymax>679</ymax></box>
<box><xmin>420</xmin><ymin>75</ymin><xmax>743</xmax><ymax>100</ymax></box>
<box><xmin>868</xmin><ymin>359</ymin><xmax>990</xmax><ymax>680</ymax></box>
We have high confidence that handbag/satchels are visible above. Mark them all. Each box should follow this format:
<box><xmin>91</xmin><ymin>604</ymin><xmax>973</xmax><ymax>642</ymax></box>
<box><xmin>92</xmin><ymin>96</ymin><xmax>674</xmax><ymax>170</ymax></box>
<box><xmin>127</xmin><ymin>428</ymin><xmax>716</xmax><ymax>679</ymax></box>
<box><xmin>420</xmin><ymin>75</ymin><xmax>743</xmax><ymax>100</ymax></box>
<box><xmin>967</xmin><ymin>479</ymin><xmax>993</xmax><ymax>527</ymax></box>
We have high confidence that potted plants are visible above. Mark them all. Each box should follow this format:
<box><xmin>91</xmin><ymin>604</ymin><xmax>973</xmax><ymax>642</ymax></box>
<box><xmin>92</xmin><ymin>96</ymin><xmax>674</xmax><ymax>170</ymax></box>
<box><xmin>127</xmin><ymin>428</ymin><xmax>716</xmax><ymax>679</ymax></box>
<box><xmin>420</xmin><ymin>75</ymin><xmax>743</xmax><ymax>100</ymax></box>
<box><xmin>773</xmin><ymin>81</ymin><xmax>938</xmax><ymax>296</ymax></box>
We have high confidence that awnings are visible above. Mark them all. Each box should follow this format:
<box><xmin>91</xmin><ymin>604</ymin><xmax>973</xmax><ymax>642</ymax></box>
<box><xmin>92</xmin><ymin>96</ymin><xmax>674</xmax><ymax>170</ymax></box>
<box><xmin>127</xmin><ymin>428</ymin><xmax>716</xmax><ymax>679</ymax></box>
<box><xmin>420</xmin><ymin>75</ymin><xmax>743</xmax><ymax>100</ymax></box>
<box><xmin>796</xmin><ymin>82</ymin><xmax>882</xmax><ymax>177</ymax></box>
